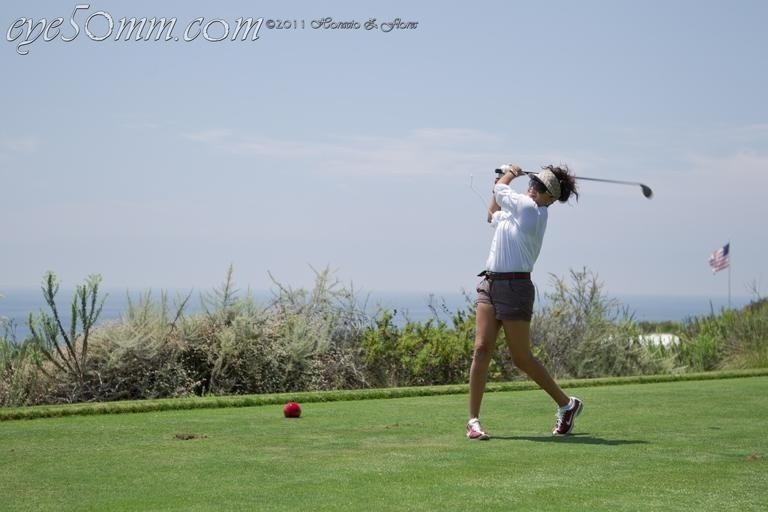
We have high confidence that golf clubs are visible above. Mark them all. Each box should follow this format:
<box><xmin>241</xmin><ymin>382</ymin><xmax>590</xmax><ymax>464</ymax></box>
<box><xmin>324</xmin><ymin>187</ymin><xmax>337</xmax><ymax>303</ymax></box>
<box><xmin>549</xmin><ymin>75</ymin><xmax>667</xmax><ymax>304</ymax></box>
<box><xmin>495</xmin><ymin>168</ymin><xmax>652</xmax><ymax>199</ymax></box>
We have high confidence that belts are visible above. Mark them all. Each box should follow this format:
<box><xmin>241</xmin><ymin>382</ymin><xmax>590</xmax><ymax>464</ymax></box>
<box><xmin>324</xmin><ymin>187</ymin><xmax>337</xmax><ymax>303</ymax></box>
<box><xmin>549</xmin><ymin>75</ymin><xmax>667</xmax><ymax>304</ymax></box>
<box><xmin>477</xmin><ymin>270</ymin><xmax>530</xmax><ymax>279</ymax></box>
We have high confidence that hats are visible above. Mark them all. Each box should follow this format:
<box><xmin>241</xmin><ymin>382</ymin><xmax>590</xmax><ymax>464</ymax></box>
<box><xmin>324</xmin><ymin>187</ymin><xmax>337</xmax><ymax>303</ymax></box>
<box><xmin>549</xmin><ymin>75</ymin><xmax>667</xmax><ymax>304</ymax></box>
<box><xmin>533</xmin><ymin>167</ymin><xmax>561</xmax><ymax>199</ymax></box>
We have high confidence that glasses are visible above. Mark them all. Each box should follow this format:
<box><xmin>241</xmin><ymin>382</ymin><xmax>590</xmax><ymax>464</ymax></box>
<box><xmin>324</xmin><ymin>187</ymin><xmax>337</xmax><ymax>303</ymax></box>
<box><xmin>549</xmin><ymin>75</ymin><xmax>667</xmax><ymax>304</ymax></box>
<box><xmin>529</xmin><ymin>177</ymin><xmax>553</xmax><ymax>196</ymax></box>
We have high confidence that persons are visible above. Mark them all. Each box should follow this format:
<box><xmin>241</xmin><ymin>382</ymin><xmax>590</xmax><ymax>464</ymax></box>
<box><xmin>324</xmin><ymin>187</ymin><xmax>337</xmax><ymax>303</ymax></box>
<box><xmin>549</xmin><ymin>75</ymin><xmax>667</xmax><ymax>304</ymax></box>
<box><xmin>463</xmin><ymin>162</ymin><xmax>584</xmax><ymax>440</ymax></box>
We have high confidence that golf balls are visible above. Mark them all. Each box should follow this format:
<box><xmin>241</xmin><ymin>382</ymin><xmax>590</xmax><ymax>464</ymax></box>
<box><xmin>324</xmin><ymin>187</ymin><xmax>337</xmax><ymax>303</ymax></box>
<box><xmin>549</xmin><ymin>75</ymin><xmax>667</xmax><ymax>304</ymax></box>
<box><xmin>284</xmin><ymin>404</ymin><xmax>301</xmax><ymax>418</ymax></box>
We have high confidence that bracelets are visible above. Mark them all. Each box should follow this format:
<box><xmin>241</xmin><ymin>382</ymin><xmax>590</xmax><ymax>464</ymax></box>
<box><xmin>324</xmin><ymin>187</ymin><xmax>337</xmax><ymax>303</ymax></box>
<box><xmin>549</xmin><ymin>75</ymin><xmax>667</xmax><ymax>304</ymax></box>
<box><xmin>510</xmin><ymin>168</ymin><xmax>519</xmax><ymax>177</ymax></box>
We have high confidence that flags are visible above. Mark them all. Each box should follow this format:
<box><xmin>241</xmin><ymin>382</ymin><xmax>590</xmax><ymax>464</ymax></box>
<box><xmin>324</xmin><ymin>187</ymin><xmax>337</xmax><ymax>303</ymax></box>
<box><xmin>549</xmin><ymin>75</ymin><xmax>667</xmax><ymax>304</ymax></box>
<box><xmin>710</xmin><ymin>244</ymin><xmax>730</xmax><ymax>276</ymax></box>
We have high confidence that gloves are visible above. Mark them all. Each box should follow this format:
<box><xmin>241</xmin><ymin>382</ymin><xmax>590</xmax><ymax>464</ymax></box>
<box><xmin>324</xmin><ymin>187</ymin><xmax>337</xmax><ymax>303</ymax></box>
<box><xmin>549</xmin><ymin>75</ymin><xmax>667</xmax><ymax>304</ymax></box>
<box><xmin>495</xmin><ymin>163</ymin><xmax>525</xmax><ymax>178</ymax></box>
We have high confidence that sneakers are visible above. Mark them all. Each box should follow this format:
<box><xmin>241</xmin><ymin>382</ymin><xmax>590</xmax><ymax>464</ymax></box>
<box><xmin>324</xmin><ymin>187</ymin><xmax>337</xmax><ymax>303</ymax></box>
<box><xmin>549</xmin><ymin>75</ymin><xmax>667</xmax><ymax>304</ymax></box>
<box><xmin>467</xmin><ymin>415</ymin><xmax>489</xmax><ymax>440</ymax></box>
<box><xmin>552</xmin><ymin>396</ymin><xmax>584</xmax><ymax>437</ymax></box>
